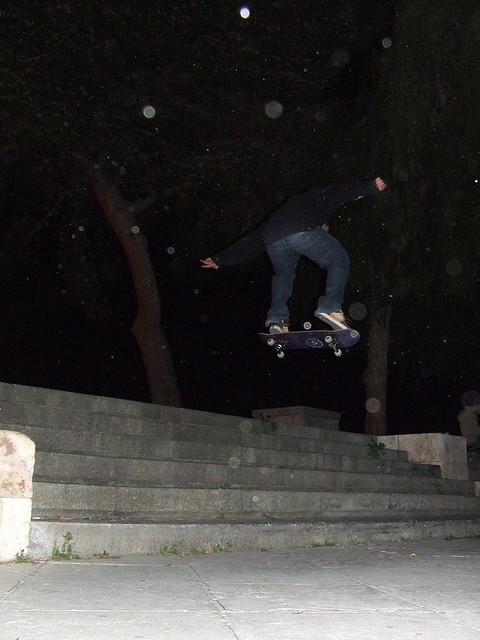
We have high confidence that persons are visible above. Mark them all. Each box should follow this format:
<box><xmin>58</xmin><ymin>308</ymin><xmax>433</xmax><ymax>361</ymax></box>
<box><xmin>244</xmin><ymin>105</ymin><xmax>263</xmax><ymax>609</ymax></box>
<box><xmin>199</xmin><ymin>175</ymin><xmax>387</xmax><ymax>334</ymax></box>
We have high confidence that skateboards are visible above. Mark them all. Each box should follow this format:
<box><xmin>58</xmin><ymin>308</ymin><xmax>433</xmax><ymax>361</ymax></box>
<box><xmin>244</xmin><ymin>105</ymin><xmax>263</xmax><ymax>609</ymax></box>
<box><xmin>258</xmin><ymin>329</ymin><xmax>361</xmax><ymax>358</ymax></box>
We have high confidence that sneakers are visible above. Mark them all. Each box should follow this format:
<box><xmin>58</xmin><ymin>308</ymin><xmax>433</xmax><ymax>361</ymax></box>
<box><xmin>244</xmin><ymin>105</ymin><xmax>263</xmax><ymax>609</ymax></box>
<box><xmin>317</xmin><ymin>310</ymin><xmax>351</xmax><ymax>329</ymax></box>
<box><xmin>269</xmin><ymin>323</ymin><xmax>289</xmax><ymax>333</ymax></box>
